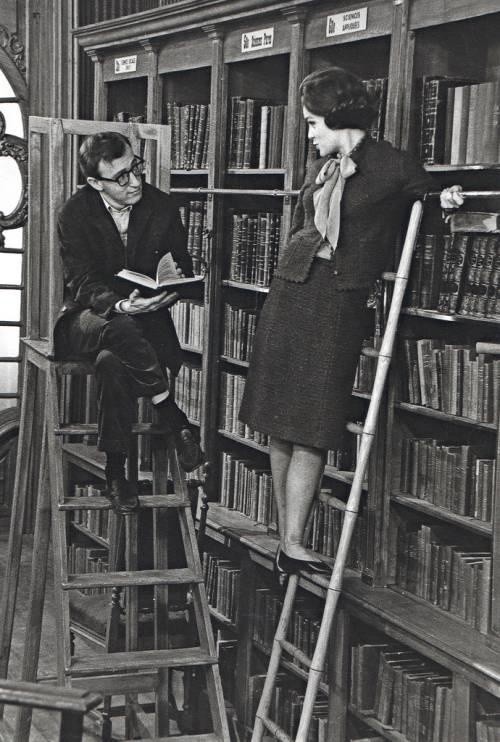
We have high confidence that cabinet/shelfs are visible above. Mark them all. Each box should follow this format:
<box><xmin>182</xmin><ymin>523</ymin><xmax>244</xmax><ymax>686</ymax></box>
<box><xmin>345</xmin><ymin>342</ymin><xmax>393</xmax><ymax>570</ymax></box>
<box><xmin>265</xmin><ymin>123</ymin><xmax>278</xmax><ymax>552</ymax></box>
<box><xmin>62</xmin><ymin>0</ymin><xmax>499</xmax><ymax>740</ymax></box>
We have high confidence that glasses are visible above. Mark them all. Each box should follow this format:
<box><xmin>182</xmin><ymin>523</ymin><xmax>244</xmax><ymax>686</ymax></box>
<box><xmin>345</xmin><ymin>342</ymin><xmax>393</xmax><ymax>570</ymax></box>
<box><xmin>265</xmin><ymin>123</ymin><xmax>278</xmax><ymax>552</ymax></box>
<box><xmin>97</xmin><ymin>154</ymin><xmax>146</xmax><ymax>186</ymax></box>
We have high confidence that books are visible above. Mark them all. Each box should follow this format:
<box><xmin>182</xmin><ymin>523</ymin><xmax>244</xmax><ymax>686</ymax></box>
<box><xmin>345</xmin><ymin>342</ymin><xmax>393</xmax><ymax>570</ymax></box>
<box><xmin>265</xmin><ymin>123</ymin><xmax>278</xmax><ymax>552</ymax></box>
<box><xmin>220</xmin><ymin>376</ymin><xmax>272</xmax><ymax>448</ymax></box>
<box><xmin>167</xmin><ymin>360</ymin><xmax>203</xmax><ymax>421</ymax></box>
<box><xmin>245</xmin><ymin>674</ymin><xmax>331</xmax><ymax>742</ymax></box>
<box><xmin>217</xmin><ymin>453</ymin><xmax>277</xmax><ymax>532</ymax></box>
<box><xmin>166</xmin><ymin>294</ymin><xmax>206</xmax><ymax>351</ymax></box>
<box><xmin>217</xmin><ymin>305</ymin><xmax>255</xmax><ymax>361</ymax></box>
<box><xmin>57</xmin><ymin>369</ymin><xmax>102</xmax><ymax>426</ymax></box>
<box><xmin>64</xmin><ymin>471</ymin><xmax>116</xmax><ymax>539</ymax></box>
<box><xmin>391</xmin><ymin>69</ymin><xmax>499</xmax><ymax>647</ymax></box>
<box><xmin>64</xmin><ymin>544</ymin><xmax>107</xmax><ymax>603</ymax></box>
<box><xmin>221</xmin><ymin>208</ymin><xmax>278</xmax><ymax>291</ymax></box>
<box><xmin>323</xmin><ymin>418</ymin><xmax>362</xmax><ymax>472</ymax></box>
<box><xmin>163</xmin><ymin>97</ymin><xmax>210</xmax><ymax>167</ymax></box>
<box><xmin>353</xmin><ymin>337</ymin><xmax>383</xmax><ymax>393</ymax></box>
<box><xmin>196</xmin><ymin>546</ymin><xmax>331</xmax><ymax>684</ymax></box>
<box><xmin>224</xmin><ymin>93</ymin><xmax>284</xmax><ymax>167</ymax></box>
<box><xmin>306</xmin><ymin>502</ymin><xmax>364</xmax><ymax>568</ymax></box>
<box><xmin>349</xmin><ymin>631</ymin><xmax>500</xmax><ymax>741</ymax></box>
<box><xmin>174</xmin><ymin>196</ymin><xmax>207</xmax><ymax>281</ymax></box>
<box><xmin>114</xmin><ymin>252</ymin><xmax>201</xmax><ymax>291</ymax></box>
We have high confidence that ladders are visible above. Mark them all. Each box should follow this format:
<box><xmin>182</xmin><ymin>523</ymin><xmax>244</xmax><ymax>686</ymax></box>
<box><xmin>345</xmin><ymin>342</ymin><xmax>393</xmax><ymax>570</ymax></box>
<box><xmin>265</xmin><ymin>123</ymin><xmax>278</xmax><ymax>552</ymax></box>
<box><xmin>0</xmin><ymin>337</ymin><xmax>233</xmax><ymax>742</ymax></box>
<box><xmin>249</xmin><ymin>201</ymin><xmax>425</xmax><ymax>742</ymax></box>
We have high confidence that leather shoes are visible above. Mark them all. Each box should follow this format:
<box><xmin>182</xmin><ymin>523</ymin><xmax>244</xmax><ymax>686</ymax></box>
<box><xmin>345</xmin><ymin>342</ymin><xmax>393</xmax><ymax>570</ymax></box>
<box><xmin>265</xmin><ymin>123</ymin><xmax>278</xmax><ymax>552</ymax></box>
<box><xmin>179</xmin><ymin>428</ymin><xmax>201</xmax><ymax>472</ymax></box>
<box><xmin>279</xmin><ymin>550</ymin><xmax>332</xmax><ymax>573</ymax></box>
<box><xmin>109</xmin><ymin>474</ymin><xmax>141</xmax><ymax>515</ymax></box>
<box><xmin>274</xmin><ymin>547</ymin><xmax>288</xmax><ymax>587</ymax></box>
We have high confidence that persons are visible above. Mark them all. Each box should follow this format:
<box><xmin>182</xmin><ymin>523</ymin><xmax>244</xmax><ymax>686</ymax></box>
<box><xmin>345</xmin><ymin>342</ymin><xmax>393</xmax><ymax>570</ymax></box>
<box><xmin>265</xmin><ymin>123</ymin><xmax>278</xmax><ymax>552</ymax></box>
<box><xmin>236</xmin><ymin>64</ymin><xmax>467</xmax><ymax>592</ymax></box>
<box><xmin>49</xmin><ymin>129</ymin><xmax>208</xmax><ymax>516</ymax></box>
